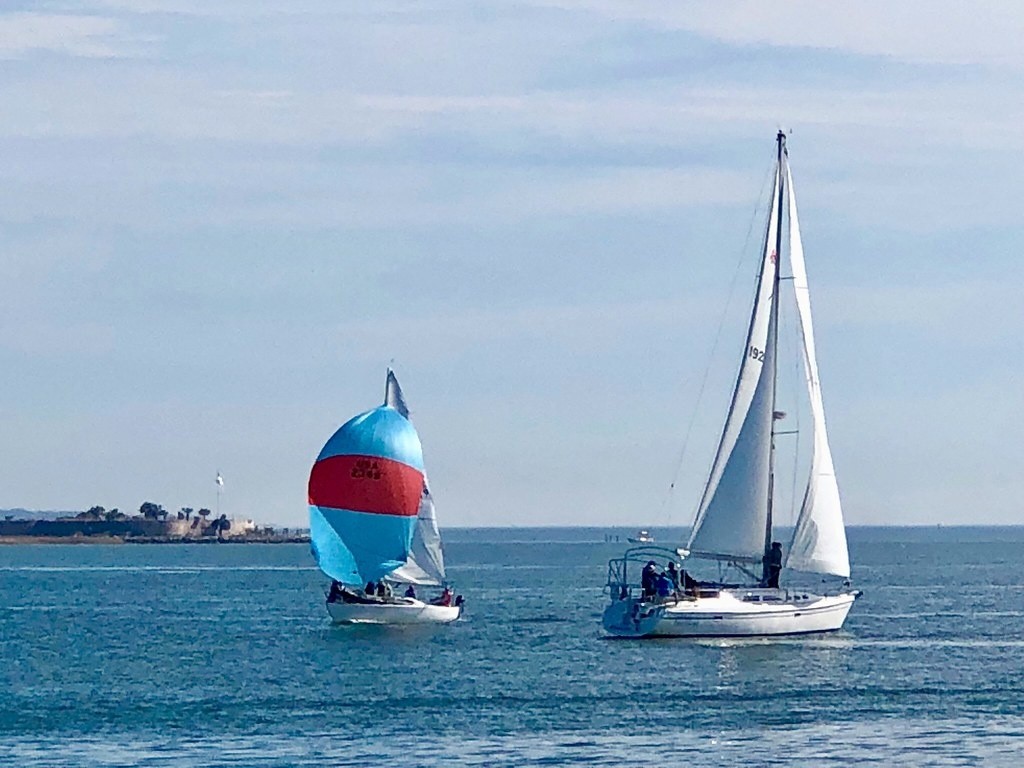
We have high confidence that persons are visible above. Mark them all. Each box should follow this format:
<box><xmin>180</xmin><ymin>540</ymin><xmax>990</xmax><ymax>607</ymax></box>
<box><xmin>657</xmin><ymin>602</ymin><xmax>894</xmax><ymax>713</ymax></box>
<box><xmin>641</xmin><ymin>560</ymin><xmax>701</xmax><ymax>597</ymax></box>
<box><xmin>330</xmin><ymin>578</ymin><xmax>393</xmax><ymax>603</ymax></box>
<box><xmin>762</xmin><ymin>542</ymin><xmax>782</xmax><ymax>588</ymax></box>
<box><xmin>430</xmin><ymin>587</ymin><xmax>454</xmax><ymax>605</ymax></box>
<box><xmin>405</xmin><ymin>584</ymin><xmax>416</xmax><ymax>602</ymax></box>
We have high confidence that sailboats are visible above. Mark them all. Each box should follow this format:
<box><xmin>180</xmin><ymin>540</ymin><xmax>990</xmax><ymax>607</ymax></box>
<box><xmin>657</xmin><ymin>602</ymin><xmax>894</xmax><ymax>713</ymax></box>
<box><xmin>306</xmin><ymin>364</ymin><xmax>467</xmax><ymax>624</ymax></box>
<box><xmin>597</xmin><ymin>126</ymin><xmax>862</xmax><ymax>642</ymax></box>
